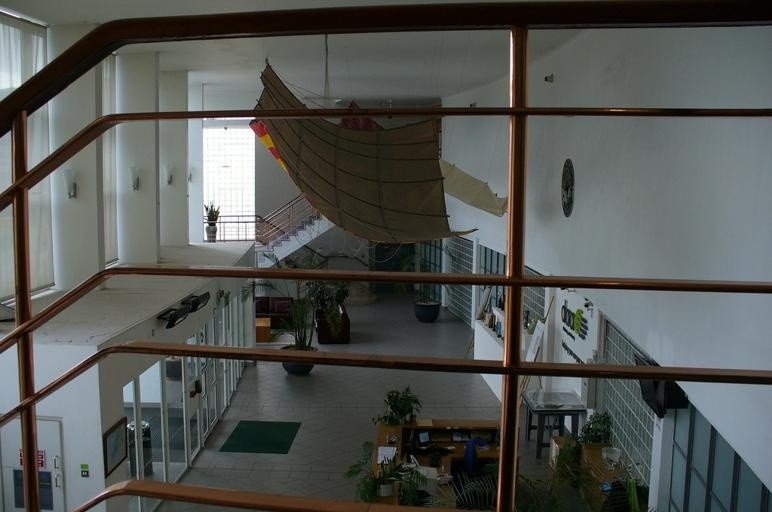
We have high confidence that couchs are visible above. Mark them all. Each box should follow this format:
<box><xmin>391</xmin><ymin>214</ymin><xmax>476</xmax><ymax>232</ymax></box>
<box><xmin>256</xmin><ymin>296</ymin><xmax>293</xmax><ymax>328</ymax></box>
<box><xmin>316</xmin><ymin>303</ymin><xmax>350</xmax><ymax>344</ymax></box>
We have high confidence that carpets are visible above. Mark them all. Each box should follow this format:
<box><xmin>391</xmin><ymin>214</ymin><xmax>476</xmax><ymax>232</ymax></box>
<box><xmin>218</xmin><ymin>419</ymin><xmax>301</xmax><ymax>455</ymax></box>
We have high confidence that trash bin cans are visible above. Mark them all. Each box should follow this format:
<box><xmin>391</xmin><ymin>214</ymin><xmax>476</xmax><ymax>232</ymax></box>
<box><xmin>127</xmin><ymin>420</ymin><xmax>153</xmax><ymax>477</ymax></box>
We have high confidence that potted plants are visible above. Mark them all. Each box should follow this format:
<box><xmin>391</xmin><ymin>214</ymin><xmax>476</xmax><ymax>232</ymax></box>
<box><xmin>344</xmin><ymin>441</ymin><xmax>420</xmax><ymax>506</ymax></box>
<box><xmin>204</xmin><ymin>200</ymin><xmax>221</xmax><ymax>244</ymax></box>
<box><xmin>242</xmin><ymin>249</ymin><xmax>349</xmax><ymax>376</ymax></box>
<box><xmin>373</xmin><ymin>387</ymin><xmax>421</xmax><ymax>426</ymax></box>
<box><xmin>399</xmin><ymin>256</ymin><xmax>449</xmax><ymax>323</ymax></box>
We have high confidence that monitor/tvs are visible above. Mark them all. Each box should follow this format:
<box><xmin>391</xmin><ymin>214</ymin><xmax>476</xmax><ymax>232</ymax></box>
<box><xmin>417</xmin><ymin>429</ymin><xmax>432</xmax><ymax>447</ymax></box>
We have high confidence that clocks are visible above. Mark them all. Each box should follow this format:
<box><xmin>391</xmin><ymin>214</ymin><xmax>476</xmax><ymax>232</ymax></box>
<box><xmin>561</xmin><ymin>158</ymin><xmax>574</xmax><ymax>217</ymax></box>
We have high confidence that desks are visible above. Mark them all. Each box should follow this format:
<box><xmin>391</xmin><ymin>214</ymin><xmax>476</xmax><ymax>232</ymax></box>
<box><xmin>522</xmin><ymin>392</ymin><xmax>587</xmax><ymax>459</ymax></box>
<box><xmin>400</xmin><ymin>440</ymin><xmax>520</xmax><ymax>510</ymax></box>
<box><xmin>575</xmin><ymin>441</ymin><xmax>635</xmax><ymax>511</ymax></box>
<box><xmin>256</xmin><ymin>317</ymin><xmax>271</xmax><ymax>341</ymax></box>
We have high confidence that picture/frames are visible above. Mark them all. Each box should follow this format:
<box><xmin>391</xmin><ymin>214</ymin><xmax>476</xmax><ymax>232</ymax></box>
<box><xmin>104</xmin><ymin>416</ymin><xmax>127</xmax><ymax>478</ymax></box>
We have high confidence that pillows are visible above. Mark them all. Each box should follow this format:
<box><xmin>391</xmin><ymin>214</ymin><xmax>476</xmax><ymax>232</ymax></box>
<box><xmin>270</xmin><ymin>300</ymin><xmax>290</xmax><ymax>313</ymax></box>
<box><xmin>257</xmin><ymin>300</ymin><xmax>269</xmax><ymax>313</ymax></box>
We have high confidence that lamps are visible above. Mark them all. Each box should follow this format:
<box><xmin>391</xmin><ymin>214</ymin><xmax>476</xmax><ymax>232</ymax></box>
<box><xmin>129</xmin><ymin>166</ymin><xmax>140</xmax><ymax>191</ymax></box>
<box><xmin>166</xmin><ymin>160</ymin><xmax>174</xmax><ymax>184</ymax></box>
<box><xmin>183</xmin><ymin>292</ymin><xmax>210</xmax><ymax>313</ymax></box>
<box><xmin>220</xmin><ymin>291</ymin><xmax>231</xmax><ymax>307</ymax></box>
<box><xmin>63</xmin><ymin>170</ymin><xmax>76</xmax><ymax>201</ymax></box>
<box><xmin>158</xmin><ymin>305</ymin><xmax>189</xmax><ymax>330</ymax></box>
